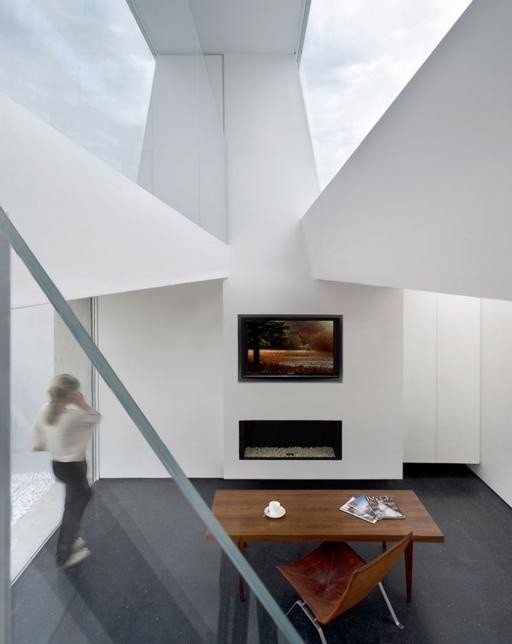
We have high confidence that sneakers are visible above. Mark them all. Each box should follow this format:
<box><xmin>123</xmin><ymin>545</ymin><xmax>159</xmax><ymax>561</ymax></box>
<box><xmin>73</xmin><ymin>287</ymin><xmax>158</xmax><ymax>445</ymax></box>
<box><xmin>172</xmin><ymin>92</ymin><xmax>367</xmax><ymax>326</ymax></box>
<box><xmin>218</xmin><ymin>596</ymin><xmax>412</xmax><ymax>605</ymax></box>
<box><xmin>70</xmin><ymin>536</ymin><xmax>87</xmax><ymax>551</ymax></box>
<box><xmin>61</xmin><ymin>547</ymin><xmax>91</xmax><ymax>569</ymax></box>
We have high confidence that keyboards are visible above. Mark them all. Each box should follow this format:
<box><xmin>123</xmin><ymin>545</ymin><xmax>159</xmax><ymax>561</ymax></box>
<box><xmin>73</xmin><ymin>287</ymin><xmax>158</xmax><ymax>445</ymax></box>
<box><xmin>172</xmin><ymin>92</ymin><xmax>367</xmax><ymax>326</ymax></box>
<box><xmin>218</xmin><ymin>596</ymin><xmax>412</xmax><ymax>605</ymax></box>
<box><xmin>244</xmin><ymin>447</ymin><xmax>335</xmax><ymax>457</ymax></box>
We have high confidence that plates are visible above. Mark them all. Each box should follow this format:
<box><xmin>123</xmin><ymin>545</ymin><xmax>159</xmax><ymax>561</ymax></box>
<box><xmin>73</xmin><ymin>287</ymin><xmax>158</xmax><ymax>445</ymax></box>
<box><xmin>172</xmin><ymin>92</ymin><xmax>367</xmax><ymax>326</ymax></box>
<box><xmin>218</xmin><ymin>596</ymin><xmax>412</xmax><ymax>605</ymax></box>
<box><xmin>264</xmin><ymin>506</ymin><xmax>286</xmax><ymax>518</ymax></box>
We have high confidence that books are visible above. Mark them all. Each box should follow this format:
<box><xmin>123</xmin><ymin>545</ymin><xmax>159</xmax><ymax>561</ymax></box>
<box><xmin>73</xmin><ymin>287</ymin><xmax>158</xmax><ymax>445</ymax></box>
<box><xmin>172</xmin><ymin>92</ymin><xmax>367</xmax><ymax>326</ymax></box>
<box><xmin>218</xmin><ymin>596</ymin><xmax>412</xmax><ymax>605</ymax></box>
<box><xmin>338</xmin><ymin>495</ymin><xmax>406</xmax><ymax>524</ymax></box>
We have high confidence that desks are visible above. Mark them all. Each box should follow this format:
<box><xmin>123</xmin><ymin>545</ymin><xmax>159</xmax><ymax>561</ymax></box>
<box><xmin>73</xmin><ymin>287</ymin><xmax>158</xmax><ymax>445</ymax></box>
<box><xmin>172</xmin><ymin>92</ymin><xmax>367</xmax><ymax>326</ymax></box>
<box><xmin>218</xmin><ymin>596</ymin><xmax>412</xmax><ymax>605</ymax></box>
<box><xmin>207</xmin><ymin>489</ymin><xmax>444</xmax><ymax>604</ymax></box>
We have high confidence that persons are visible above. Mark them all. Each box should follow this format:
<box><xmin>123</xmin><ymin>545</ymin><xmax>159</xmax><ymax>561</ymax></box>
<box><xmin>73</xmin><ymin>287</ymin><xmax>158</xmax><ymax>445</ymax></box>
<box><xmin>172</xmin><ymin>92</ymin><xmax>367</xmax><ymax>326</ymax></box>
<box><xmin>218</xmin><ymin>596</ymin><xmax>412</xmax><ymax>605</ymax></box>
<box><xmin>30</xmin><ymin>372</ymin><xmax>102</xmax><ymax>570</ymax></box>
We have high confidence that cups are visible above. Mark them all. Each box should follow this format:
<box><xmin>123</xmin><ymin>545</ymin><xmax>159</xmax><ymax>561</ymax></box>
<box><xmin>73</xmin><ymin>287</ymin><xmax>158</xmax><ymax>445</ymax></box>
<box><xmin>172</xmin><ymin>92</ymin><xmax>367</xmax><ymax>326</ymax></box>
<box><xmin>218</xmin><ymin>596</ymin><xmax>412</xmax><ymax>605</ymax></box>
<box><xmin>269</xmin><ymin>500</ymin><xmax>280</xmax><ymax>515</ymax></box>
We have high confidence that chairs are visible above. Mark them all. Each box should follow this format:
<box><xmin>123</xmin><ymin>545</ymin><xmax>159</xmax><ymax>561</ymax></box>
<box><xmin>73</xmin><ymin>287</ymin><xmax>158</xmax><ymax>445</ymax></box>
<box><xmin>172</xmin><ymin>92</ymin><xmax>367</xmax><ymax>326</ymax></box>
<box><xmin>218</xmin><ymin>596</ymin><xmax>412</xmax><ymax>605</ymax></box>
<box><xmin>275</xmin><ymin>532</ymin><xmax>413</xmax><ymax>644</ymax></box>
